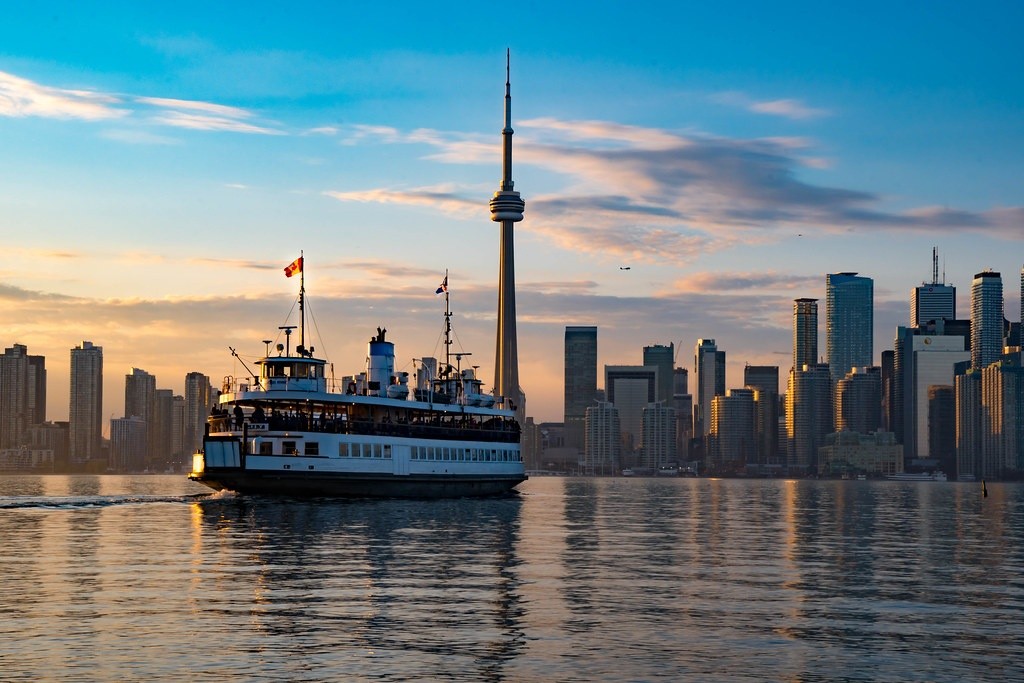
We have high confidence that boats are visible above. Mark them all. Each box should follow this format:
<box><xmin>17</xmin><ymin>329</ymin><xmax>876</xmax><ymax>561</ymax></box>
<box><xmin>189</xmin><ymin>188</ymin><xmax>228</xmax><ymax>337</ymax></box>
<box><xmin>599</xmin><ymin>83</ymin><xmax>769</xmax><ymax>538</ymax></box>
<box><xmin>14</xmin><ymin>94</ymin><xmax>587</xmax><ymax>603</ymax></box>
<box><xmin>884</xmin><ymin>470</ymin><xmax>948</xmax><ymax>482</ymax></box>
<box><xmin>184</xmin><ymin>248</ymin><xmax>530</xmax><ymax>498</ymax></box>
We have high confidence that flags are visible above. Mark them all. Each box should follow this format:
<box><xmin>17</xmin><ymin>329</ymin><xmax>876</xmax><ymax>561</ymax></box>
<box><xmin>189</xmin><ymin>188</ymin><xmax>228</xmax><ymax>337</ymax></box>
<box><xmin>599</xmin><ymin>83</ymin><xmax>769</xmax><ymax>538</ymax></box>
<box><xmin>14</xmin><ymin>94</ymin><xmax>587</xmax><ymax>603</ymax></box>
<box><xmin>284</xmin><ymin>257</ymin><xmax>302</xmax><ymax>277</ymax></box>
<box><xmin>436</xmin><ymin>276</ymin><xmax>447</xmax><ymax>294</ymax></box>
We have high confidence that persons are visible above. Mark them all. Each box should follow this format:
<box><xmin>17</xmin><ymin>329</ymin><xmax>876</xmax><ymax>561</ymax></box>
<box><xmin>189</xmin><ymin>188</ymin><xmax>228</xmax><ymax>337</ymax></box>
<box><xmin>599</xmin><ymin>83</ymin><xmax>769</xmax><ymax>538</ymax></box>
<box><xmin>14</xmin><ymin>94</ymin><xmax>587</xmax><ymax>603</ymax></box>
<box><xmin>211</xmin><ymin>403</ymin><xmax>221</xmax><ymax>432</ymax></box>
<box><xmin>252</xmin><ymin>404</ymin><xmax>333</xmax><ymax>428</ymax></box>
<box><xmin>380</xmin><ymin>416</ymin><xmax>393</xmax><ymax>430</ymax></box>
<box><xmin>234</xmin><ymin>402</ymin><xmax>244</xmax><ymax>430</ymax></box>
